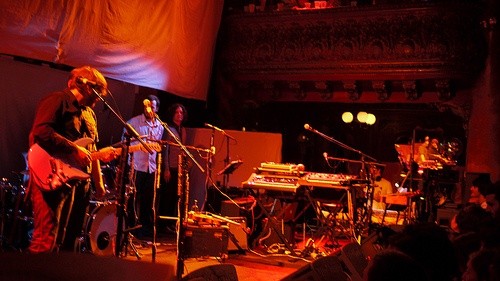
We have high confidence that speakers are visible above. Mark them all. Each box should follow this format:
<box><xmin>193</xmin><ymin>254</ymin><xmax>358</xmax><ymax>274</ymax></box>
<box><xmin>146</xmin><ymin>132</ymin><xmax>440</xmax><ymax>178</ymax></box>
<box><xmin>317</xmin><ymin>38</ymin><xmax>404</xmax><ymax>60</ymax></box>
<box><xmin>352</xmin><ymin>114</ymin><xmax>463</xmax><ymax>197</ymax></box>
<box><xmin>0</xmin><ymin>252</ymin><xmax>175</xmax><ymax>281</ymax></box>
<box><xmin>182</xmin><ymin>263</ymin><xmax>238</xmax><ymax>281</ymax></box>
<box><xmin>280</xmin><ymin>232</ymin><xmax>385</xmax><ymax>281</ymax></box>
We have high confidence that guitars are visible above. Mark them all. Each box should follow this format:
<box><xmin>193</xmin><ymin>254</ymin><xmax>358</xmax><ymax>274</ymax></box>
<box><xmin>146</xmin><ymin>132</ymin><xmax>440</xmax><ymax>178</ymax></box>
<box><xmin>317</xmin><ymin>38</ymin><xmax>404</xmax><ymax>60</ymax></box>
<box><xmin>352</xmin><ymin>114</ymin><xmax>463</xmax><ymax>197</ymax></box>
<box><xmin>27</xmin><ymin>138</ymin><xmax>161</xmax><ymax>191</ymax></box>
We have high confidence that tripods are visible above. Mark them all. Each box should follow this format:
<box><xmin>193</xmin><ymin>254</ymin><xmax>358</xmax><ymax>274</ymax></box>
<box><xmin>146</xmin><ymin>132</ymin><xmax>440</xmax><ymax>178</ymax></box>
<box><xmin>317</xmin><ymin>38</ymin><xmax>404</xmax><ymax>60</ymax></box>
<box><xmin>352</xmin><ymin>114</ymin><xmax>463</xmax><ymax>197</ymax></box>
<box><xmin>119</xmin><ymin>230</ymin><xmax>144</xmax><ymax>259</ymax></box>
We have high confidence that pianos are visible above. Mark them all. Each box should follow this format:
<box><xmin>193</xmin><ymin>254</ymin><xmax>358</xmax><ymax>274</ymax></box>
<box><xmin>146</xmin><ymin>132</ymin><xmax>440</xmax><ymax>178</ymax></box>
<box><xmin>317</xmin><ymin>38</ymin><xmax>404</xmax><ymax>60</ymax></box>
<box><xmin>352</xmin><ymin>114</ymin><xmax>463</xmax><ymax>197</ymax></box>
<box><xmin>242</xmin><ymin>162</ymin><xmax>358</xmax><ymax>204</ymax></box>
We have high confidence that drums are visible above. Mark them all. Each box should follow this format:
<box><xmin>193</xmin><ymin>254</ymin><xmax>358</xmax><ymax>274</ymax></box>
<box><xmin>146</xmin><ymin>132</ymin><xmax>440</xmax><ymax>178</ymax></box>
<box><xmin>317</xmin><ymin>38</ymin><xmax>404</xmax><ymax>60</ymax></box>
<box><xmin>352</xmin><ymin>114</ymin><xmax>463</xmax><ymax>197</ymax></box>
<box><xmin>82</xmin><ymin>202</ymin><xmax>130</xmax><ymax>257</ymax></box>
<box><xmin>90</xmin><ymin>166</ymin><xmax>116</xmax><ymax>192</ymax></box>
<box><xmin>6</xmin><ymin>210</ymin><xmax>34</xmax><ymax>249</ymax></box>
<box><xmin>0</xmin><ymin>178</ymin><xmax>26</xmax><ymax>208</ymax></box>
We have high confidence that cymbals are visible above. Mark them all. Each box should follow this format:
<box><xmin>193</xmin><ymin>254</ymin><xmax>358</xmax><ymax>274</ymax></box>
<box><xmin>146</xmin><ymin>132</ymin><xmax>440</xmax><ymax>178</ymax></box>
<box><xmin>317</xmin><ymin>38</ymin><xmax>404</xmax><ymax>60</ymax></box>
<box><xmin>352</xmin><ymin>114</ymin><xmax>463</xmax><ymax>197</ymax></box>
<box><xmin>12</xmin><ymin>170</ymin><xmax>30</xmax><ymax>175</ymax></box>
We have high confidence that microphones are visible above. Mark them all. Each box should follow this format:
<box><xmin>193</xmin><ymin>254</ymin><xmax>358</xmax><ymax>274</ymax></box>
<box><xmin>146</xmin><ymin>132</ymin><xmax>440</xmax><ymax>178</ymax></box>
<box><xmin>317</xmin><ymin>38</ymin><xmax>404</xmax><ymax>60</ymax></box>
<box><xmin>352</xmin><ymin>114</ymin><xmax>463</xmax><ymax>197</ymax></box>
<box><xmin>143</xmin><ymin>99</ymin><xmax>155</xmax><ymax>125</ymax></box>
<box><xmin>204</xmin><ymin>122</ymin><xmax>223</xmax><ymax>132</ymax></box>
<box><xmin>304</xmin><ymin>123</ymin><xmax>318</xmax><ymax>133</ymax></box>
<box><xmin>75</xmin><ymin>75</ymin><xmax>105</xmax><ymax>90</ymax></box>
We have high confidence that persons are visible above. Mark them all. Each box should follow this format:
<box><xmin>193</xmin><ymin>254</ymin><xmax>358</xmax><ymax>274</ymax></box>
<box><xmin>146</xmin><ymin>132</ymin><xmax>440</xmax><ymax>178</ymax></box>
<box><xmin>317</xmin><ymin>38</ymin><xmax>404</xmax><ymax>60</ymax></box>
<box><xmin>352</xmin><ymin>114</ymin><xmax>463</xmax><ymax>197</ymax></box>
<box><xmin>419</xmin><ymin>136</ymin><xmax>453</xmax><ymax>166</ymax></box>
<box><xmin>160</xmin><ymin>103</ymin><xmax>187</xmax><ymax>234</ymax></box>
<box><xmin>23</xmin><ymin>66</ymin><xmax>107</xmax><ymax>254</ymax></box>
<box><xmin>363</xmin><ymin>163</ymin><xmax>392</xmax><ymax>236</ymax></box>
<box><xmin>121</xmin><ymin>95</ymin><xmax>163</xmax><ymax>239</ymax></box>
<box><xmin>402</xmin><ymin>161</ymin><xmax>420</xmax><ymax>192</ymax></box>
<box><xmin>360</xmin><ymin>176</ymin><xmax>500</xmax><ymax>281</ymax></box>
